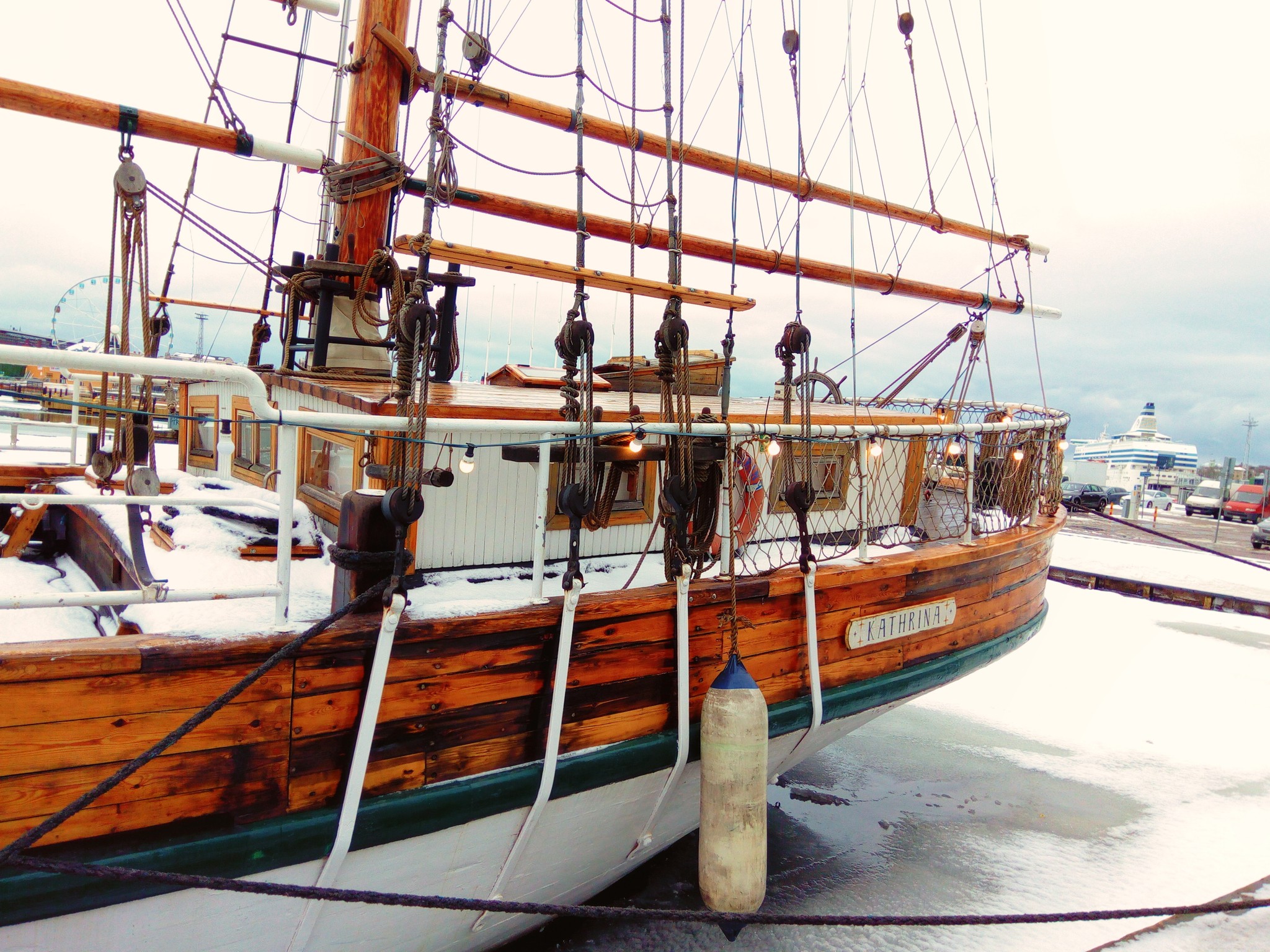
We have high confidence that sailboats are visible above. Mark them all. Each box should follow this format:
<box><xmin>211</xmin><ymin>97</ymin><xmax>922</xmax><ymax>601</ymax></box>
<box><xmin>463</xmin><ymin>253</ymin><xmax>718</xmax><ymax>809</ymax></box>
<box><xmin>1</xmin><ymin>1</ymin><xmax>1070</xmax><ymax>952</ymax></box>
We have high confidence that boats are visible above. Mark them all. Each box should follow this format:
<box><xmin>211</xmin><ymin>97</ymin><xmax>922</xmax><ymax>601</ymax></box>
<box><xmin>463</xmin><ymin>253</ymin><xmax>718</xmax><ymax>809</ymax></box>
<box><xmin>1070</xmin><ymin>402</ymin><xmax>1198</xmax><ymax>505</ymax></box>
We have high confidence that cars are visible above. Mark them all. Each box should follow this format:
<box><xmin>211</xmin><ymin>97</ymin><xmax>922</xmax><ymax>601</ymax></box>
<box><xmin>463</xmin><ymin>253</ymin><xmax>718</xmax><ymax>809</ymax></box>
<box><xmin>1060</xmin><ymin>480</ymin><xmax>1107</xmax><ymax>513</ymax></box>
<box><xmin>1101</xmin><ymin>485</ymin><xmax>1131</xmax><ymax>505</ymax></box>
<box><xmin>1119</xmin><ymin>489</ymin><xmax>1172</xmax><ymax>511</ymax></box>
<box><xmin>1251</xmin><ymin>516</ymin><xmax>1270</xmax><ymax>549</ymax></box>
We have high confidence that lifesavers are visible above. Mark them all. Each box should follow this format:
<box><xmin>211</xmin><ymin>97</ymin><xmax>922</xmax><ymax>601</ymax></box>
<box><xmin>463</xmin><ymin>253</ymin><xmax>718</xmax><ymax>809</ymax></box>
<box><xmin>683</xmin><ymin>445</ymin><xmax>765</xmax><ymax>555</ymax></box>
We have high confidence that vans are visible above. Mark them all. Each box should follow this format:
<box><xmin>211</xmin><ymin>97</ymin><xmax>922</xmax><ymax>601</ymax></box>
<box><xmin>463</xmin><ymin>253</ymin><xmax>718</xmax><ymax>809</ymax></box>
<box><xmin>1185</xmin><ymin>479</ymin><xmax>1242</xmax><ymax>519</ymax></box>
<box><xmin>1224</xmin><ymin>484</ymin><xmax>1270</xmax><ymax>525</ymax></box>
